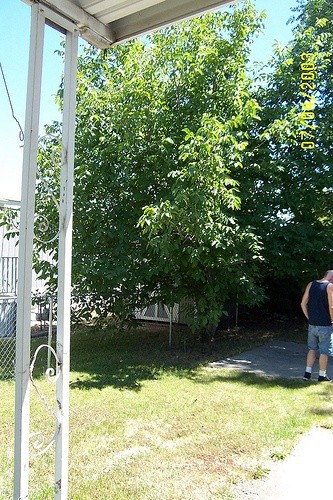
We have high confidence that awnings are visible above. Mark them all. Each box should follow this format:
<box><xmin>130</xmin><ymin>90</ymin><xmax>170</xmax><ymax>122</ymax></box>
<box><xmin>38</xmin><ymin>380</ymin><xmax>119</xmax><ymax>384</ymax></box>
<box><xmin>12</xmin><ymin>0</ymin><xmax>241</xmax><ymax>499</ymax></box>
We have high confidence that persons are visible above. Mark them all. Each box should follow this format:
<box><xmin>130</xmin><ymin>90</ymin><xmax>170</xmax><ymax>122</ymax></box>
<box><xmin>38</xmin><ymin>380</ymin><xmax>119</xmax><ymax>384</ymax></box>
<box><xmin>300</xmin><ymin>269</ymin><xmax>332</xmax><ymax>383</ymax></box>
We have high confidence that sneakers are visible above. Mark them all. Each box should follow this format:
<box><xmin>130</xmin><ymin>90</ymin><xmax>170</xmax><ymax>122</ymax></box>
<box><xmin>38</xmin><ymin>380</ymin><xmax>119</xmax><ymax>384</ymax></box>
<box><xmin>318</xmin><ymin>376</ymin><xmax>332</xmax><ymax>382</ymax></box>
<box><xmin>303</xmin><ymin>372</ymin><xmax>311</xmax><ymax>380</ymax></box>
